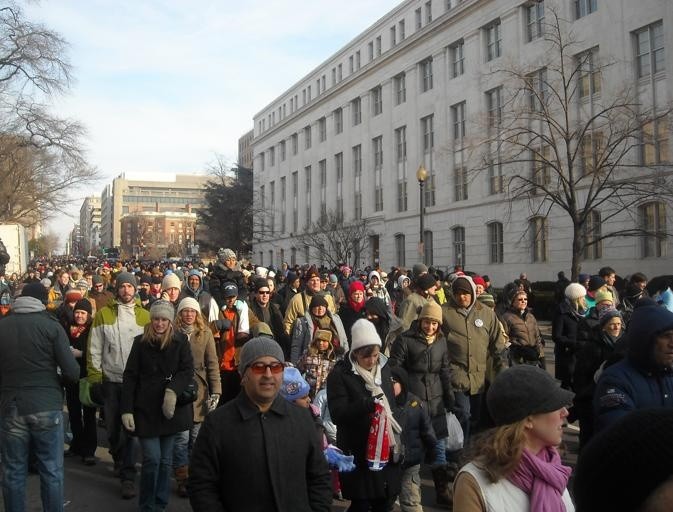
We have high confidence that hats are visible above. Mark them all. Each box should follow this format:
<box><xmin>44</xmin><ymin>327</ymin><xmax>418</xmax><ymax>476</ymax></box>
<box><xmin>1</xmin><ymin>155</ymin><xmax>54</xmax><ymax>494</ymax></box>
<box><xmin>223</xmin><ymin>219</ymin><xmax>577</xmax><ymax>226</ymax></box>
<box><xmin>328</xmin><ymin>263</ymin><xmax>437</xmax><ymax>292</ymax></box>
<box><xmin>486</xmin><ymin>364</ymin><xmax>575</xmax><ymax>426</ymax></box>
<box><xmin>309</xmin><ymin>328</ymin><xmax>337</xmax><ymax>360</ymax></box>
<box><xmin>506</xmin><ymin>288</ymin><xmax>527</xmax><ymax>308</ymax></box>
<box><xmin>473</xmin><ymin>277</ymin><xmax>486</xmax><ymax>289</ymax></box>
<box><xmin>418</xmin><ymin>302</ymin><xmax>442</xmax><ymax>328</ymax></box>
<box><xmin>20</xmin><ymin>272</ymin><xmax>56</xmax><ymax>307</ymax></box>
<box><xmin>565</xmin><ymin>276</ymin><xmax>623</xmax><ymax>330</ymax></box>
<box><xmin>65</xmin><ymin>270</ymin><xmax>204</xmax><ymax>322</ymax></box>
<box><xmin>240</xmin><ymin>337</ymin><xmax>284</xmax><ymax>377</ymax></box>
<box><xmin>453</xmin><ymin>278</ymin><xmax>472</xmax><ymax>296</ymax></box>
<box><xmin>217</xmin><ymin>248</ymin><xmax>238</xmax><ymax>264</ymax></box>
<box><xmin>351</xmin><ymin>319</ymin><xmax>383</xmax><ymax>354</ymax></box>
<box><xmin>220</xmin><ymin>267</ymin><xmax>327</xmax><ymax>311</ymax></box>
<box><xmin>278</xmin><ymin>366</ymin><xmax>309</xmax><ymax>402</ymax></box>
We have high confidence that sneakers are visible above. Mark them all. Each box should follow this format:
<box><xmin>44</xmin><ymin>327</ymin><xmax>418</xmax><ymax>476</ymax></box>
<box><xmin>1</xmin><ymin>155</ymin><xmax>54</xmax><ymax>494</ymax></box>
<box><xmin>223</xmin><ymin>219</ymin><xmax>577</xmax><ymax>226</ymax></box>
<box><xmin>114</xmin><ymin>460</ymin><xmax>136</xmax><ymax>500</ymax></box>
<box><xmin>64</xmin><ymin>450</ymin><xmax>95</xmax><ymax>466</ymax></box>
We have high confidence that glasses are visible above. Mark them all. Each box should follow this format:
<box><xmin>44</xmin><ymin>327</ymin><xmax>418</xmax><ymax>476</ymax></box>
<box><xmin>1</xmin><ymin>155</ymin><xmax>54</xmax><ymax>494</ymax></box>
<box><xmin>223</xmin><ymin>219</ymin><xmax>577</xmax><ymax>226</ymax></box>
<box><xmin>247</xmin><ymin>362</ymin><xmax>283</xmax><ymax>375</ymax></box>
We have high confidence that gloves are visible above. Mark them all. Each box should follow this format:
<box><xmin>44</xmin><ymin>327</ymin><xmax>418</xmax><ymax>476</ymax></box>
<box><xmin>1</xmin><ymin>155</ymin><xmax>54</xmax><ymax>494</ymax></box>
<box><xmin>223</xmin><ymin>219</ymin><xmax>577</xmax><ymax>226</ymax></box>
<box><xmin>162</xmin><ymin>390</ymin><xmax>176</xmax><ymax>420</ymax></box>
<box><xmin>91</xmin><ymin>383</ymin><xmax>104</xmax><ymax>405</ymax></box>
<box><xmin>206</xmin><ymin>394</ymin><xmax>220</xmax><ymax>415</ymax></box>
<box><xmin>121</xmin><ymin>413</ymin><xmax>135</xmax><ymax>432</ymax></box>
<box><xmin>367</xmin><ymin>394</ymin><xmax>383</xmax><ymax>412</ymax></box>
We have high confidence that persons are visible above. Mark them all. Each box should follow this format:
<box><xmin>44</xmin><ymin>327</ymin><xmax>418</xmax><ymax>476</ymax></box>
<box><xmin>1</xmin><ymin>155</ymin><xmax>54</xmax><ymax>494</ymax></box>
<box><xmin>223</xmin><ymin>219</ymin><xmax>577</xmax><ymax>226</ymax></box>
<box><xmin>1</xmin><ymin>247</ymin><xmax>673</xmax><ymax>511</ymax></box>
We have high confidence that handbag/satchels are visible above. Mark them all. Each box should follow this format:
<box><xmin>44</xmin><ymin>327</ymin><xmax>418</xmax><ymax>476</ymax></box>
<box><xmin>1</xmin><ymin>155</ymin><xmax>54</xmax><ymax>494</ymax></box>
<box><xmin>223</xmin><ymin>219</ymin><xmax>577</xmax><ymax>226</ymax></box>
<box><xmin>79</xmin><ymin>376</ymin><xmax>105</xmax><ymax>407</ymax></box>
<box><xmin>177</xmin><ymin>380</ymin><xmax>198</xmax><ymax>406</ymax></box>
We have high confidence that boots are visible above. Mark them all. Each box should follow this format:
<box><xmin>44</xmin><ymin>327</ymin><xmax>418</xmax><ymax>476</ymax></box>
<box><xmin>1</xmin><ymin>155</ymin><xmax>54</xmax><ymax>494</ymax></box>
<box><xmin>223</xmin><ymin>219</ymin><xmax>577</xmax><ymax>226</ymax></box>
<box><xmin>432</xmin><ymin>464</ymin><xmax>454</xmax><ymax>508</ymax></box>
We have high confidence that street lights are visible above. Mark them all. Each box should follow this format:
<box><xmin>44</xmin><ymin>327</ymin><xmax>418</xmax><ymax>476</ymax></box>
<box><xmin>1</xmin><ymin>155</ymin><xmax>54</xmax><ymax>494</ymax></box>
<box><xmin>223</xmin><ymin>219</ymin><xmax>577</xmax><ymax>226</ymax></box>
<box><xmin>416</xmin><ymin>163</ymin><xmax>429</xmax><ymax>266</ymax></box>
